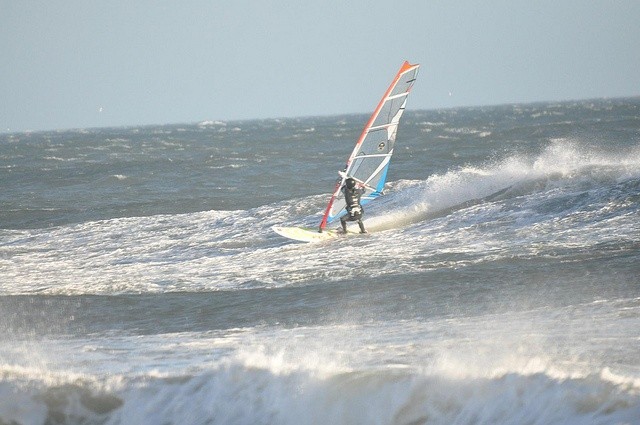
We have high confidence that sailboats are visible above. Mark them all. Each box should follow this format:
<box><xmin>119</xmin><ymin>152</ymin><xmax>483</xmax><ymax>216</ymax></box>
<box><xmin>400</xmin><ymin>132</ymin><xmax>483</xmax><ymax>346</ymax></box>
<box><xmin>270</xmin><ymin>59</ymin><xmax>420</xmax><ymax>243</ymax></box>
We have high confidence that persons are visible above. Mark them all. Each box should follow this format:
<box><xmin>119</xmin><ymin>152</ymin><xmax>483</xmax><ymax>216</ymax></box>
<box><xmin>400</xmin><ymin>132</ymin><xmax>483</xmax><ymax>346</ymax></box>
<box><xmin>336</xmin><ymin>177</ymin><xmax>367</xmax><ymax>235</ymax></box>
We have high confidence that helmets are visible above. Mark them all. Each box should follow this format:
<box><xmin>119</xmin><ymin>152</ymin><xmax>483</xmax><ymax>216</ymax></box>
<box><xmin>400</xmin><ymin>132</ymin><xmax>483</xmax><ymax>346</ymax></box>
<box><xmin>346</xmin><ymin>178</ymin><xmax>355</xmax><ymax>188</ymax></box>
